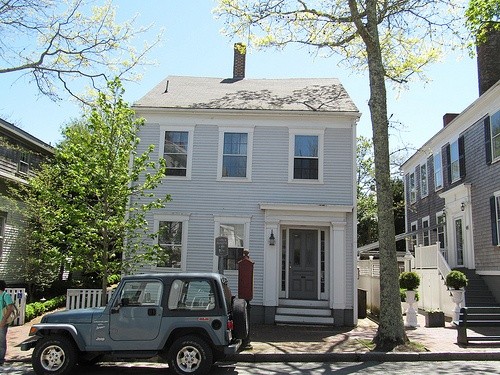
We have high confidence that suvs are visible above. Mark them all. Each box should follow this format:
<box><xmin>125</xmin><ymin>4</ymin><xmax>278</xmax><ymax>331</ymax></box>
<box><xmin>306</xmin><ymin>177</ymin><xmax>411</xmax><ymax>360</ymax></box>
<box><xmin>20</xmin><ymin>271</ymin><xmax>249</xmax><ymax>375</ymax></box>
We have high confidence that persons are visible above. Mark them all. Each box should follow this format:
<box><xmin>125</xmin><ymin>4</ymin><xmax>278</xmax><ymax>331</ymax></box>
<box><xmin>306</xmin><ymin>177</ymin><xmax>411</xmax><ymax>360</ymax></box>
<box><xmin>0</xmin><ymin>280</ymin><xmax>15</xmax><ymax>366</ymax></box>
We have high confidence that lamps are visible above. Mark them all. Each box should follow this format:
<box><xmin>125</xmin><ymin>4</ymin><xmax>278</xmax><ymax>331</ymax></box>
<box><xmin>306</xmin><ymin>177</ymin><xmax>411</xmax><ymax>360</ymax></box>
<box><xmin>442</xmin><ymin>210</ymin><xmax>446</xmax><ymax>217</ymax></box>
<box><xmin>269</xmin><ymin>231</ymin><xmax>276</xmax><ymax>246</ymax></box>
<box><xmin>461</xmin><ymin>202</ymin><xmax>465</xmax><ymax>211</ymax></box>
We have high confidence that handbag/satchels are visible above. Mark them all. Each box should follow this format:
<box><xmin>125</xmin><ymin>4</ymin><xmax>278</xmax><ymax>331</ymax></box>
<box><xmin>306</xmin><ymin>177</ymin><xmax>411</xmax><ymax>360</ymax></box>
<box><xmin>2</xmin><ymin>292</ymin><xmax>18</xmax><ymax>325</ymax></box>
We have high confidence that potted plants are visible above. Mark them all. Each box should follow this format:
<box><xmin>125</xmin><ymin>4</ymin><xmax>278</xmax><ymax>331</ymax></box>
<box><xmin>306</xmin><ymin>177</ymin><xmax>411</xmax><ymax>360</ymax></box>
<box><xmin>445</xmin><ymin>271</ymin><xmax>469</xmax><ymax>321</ymax></box>
<box><xmin>399</xmin><ymin>272</ymin><xmax>419</xmax><ymax>325</ymax></box>
<box><xmin>425</xmin><ymin>307</ymin><xmax>445</xmax><ymax>326</ymax></box>
